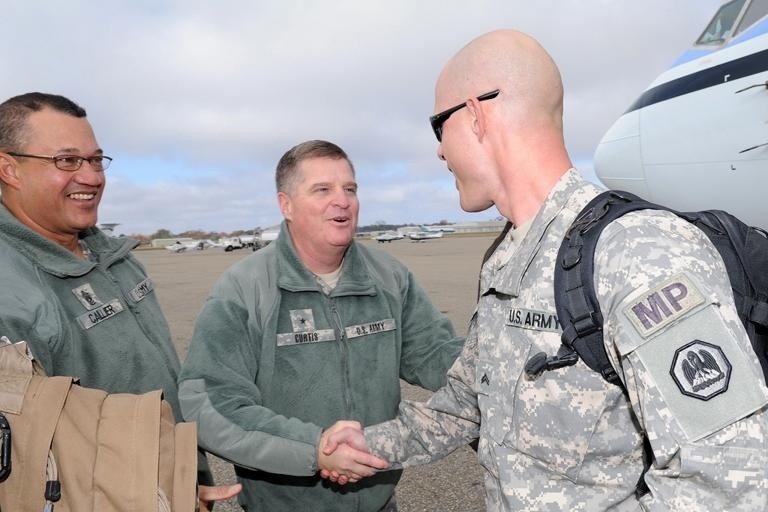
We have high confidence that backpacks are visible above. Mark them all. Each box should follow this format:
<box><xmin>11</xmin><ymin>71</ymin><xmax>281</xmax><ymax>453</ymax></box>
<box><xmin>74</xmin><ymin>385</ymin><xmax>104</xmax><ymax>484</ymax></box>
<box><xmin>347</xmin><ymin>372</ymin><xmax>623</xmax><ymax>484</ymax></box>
<box><xmin>553</xmin><ymin>188</ymin><xmax>767</xmax><ymax>389</ymax></box>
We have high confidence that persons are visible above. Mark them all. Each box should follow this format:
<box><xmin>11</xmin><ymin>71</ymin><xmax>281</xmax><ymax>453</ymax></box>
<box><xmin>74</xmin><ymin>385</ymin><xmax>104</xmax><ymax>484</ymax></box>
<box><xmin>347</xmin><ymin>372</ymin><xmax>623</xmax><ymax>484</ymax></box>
<box><xmin>174</xmin><ymin>136</ymin><xmax>482</xmax><ymax>512</ymax></box>
<box><xmin>0</xmin><ymin>89</ymin><xmax>244</xmax><ymax>511</ymax></box>
<box><xmin>316</xmin><ymin>25</ymin><xmax>768</xmax><ymax>511</ymax></box>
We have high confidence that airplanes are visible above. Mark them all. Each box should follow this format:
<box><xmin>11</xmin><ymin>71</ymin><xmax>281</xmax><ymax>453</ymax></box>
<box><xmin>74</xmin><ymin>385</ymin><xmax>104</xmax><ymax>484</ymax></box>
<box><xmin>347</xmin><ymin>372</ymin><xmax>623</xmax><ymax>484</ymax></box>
<box><xmin>592</xmin><ymin>1</ymin><xmax>768</xmax><ymax>240</ymax></box>
<box><xmin>165</xmin><ymin>224</ymin><xmax>456</xmax><ymax>254</ymax></box>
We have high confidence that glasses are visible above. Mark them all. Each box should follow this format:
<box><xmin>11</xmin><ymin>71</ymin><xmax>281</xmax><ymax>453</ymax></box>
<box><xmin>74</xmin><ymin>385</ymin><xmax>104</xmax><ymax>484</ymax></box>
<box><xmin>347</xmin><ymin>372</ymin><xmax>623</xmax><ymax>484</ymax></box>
<box><xmin>7</xmin><ymin>152</ymin><xmax>113</xmax><ymax>173</ymax></box>
<box><xmin>429</xmin><ymin>88</ymin><xmax>501</xmax><ymax>143</ymax></box>
